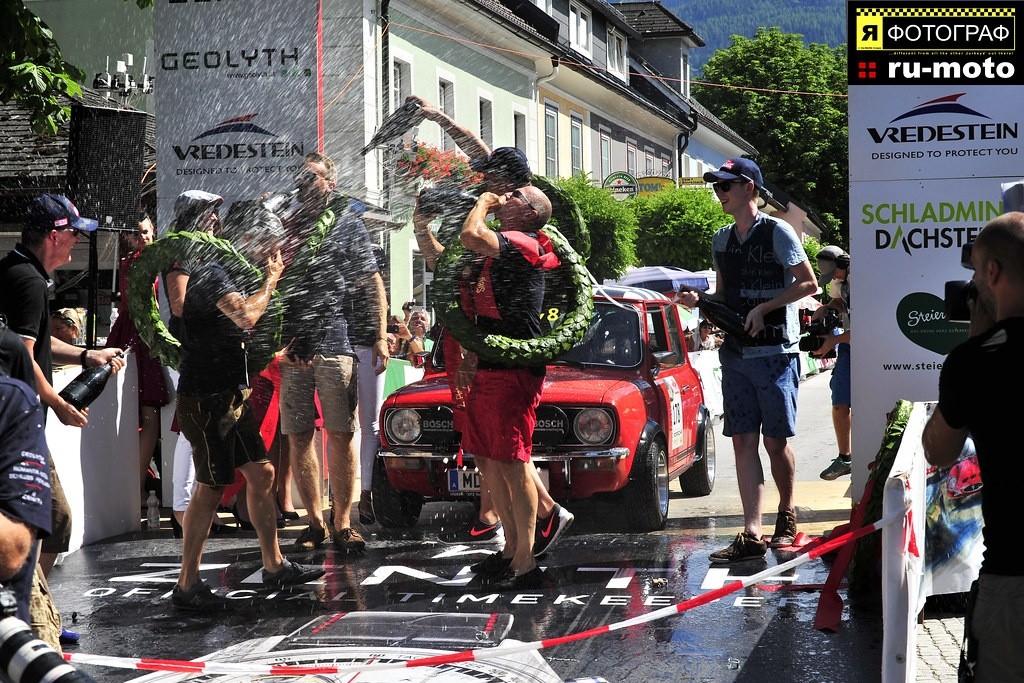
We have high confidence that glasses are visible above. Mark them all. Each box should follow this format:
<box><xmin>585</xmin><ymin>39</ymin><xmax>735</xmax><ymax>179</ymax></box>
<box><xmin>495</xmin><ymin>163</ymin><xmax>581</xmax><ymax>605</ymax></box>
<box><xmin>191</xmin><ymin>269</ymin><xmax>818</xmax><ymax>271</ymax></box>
<box><xmin>45</xmin><ymin>227</ymin><xmax>79</xmax><ymax>237</ymax></box>
<box><xmin>512</xmin><ymin>189</ymin><xmax>540</xmax><ymax>220</ymax></box>
<box><xmin>293</xmin><ymin>170</ymin><xmax>329</xmax><ymax>184</ymax></box>
<box><xmin>49</xmin><ymin>312</ymin><xmax>75</xmax><ymax>325</ymax></box>
<box><xmin>713</xmin><ymin>180</ymin><xmax>743</xmax><ymax>194</ymax></box>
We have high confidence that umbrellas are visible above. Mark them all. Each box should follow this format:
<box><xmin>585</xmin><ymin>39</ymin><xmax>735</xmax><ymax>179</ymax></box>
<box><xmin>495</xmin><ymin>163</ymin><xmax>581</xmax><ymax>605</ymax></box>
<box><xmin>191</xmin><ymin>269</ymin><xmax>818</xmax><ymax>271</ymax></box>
<box><xmin>604</xmin><ymin>266</ymin><xmax>717</xmax><ymax>335</ymax></box>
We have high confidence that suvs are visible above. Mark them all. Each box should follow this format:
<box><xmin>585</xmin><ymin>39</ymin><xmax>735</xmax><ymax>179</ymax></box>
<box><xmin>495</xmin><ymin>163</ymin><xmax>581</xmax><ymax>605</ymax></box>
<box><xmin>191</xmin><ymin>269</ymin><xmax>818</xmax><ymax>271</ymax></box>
<box><xmin>372</xmin><ymin>284</ymin><xmax>717</xmax><ymax>531</ymax></box>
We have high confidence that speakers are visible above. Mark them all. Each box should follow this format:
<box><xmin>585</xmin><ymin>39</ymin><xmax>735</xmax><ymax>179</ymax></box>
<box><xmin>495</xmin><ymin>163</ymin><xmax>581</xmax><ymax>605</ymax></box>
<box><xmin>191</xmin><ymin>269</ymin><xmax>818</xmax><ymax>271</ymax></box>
<box><xmin>66</xmin><ymin>104</ymin><xmax>149</xmax><ymax>234</ymax></box>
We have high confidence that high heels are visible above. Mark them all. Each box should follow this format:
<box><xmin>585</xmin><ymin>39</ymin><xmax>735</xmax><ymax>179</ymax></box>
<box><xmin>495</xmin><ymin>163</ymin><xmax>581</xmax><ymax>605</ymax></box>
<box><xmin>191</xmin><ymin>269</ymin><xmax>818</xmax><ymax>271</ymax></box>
<box><xmin>276</xmin><ymin>517</ymin><xmax>286</xmax><ymax>528</ymax></box>
<box><xmin>358</xmin><ymin>502</ymin><xmax>376</xmax><ymax>526</ymax></box>
<box><xmin>211</xmin><ymin>521</ymin><xmax>237</xmax><ymax>534</ymax></box>
<box><xmin>232</xmin><ymin>504</ymin><xmax>256</xmax><ymax>530</ymax></box>
<box><xmin>330</xmin><ymin>508</ymin><xmax>335</xmax><ymax>525</ymax></box>
<box><xmin>276</xmin><ymin>492</ymin><xmax>300</xmax><ymax>519</ymax></box>
<box><xmin>170</xmin><ymin>513</ymin><xmax>183</xmax><ymax>539</ymax></box>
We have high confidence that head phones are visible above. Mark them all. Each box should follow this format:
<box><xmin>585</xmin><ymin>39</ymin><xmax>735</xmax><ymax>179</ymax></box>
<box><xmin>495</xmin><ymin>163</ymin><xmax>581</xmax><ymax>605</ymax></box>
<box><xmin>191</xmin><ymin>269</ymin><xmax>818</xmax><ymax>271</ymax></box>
<box><xmin>816</xmin><ymin>253</ymin><xmax>850</xmax><ymax>269</ymax></box>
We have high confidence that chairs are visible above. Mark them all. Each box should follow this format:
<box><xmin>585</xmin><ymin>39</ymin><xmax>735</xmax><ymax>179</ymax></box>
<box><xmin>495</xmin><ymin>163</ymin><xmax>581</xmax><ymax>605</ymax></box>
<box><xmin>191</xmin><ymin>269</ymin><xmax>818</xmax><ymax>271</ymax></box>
<box><xmin>584</xmin><ymin>310</ymin><xmax>639</xmax><ymax>367</ymax></box>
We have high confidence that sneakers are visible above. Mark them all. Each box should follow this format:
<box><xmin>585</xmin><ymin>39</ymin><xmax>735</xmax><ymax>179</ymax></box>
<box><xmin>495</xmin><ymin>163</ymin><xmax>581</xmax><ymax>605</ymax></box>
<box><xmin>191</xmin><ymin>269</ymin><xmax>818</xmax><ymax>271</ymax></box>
<box><xmin>262</xmin><ymin>556</ymin><xmax>326</xmax><ymax>591</ymax></box>
<box><xmin>438</xmin><ymin>501</ymin><xmax>573</xmax><ymax>592</ymax></box>
<box><xmin>172</xmin><ymin>580</ymin><xmax>230</xmax><ymax>610</ymax></box>
<box><xmin>709</xmin><ymin>532</ymin><xmax>767</xmax><ymax>563</ymax></box>
<box><xmin>770</xmin><ymin>505</ymin><xmax>797</xmax><ymax>547</ymax></box>
<box><xmin>295</xmin><ymin>522</ymin><xmax>330</xmax><ymax>552</ymax></box>
<box><xmin>334</xmin><ymin>528</ymin><xmax>367</xmax><ymax>549</ymax></box>
<box><xmin>820</xmin><ymin>457</ymin><xmax>853</xmax><ymax>481</ymax></box>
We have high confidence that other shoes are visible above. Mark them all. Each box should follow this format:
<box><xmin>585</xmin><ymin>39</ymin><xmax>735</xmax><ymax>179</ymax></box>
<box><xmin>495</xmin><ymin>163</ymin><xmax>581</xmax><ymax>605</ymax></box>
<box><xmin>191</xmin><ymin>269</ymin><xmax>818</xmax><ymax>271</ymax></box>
<box><xmin>59</xmin><ymin>630</ymin><xmax>80</xmax><ymax>645</ymax></box>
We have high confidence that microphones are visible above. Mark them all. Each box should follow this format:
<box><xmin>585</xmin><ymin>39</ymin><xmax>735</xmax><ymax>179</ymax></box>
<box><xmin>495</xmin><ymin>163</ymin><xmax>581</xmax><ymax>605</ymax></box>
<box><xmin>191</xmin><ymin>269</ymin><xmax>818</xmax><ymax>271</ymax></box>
<box><xmin>458</xmin><ymin>193</ymin><xmax>478</xmax><ymax>205</ymax></box>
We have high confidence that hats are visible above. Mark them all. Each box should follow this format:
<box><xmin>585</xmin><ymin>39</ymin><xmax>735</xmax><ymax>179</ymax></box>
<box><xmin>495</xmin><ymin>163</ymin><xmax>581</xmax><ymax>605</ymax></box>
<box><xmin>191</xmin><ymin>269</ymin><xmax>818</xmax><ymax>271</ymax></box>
<box><xmin>703</xmin><ymin>158</ymin><xmax>764</xmax><ymax>190</ymax></box>
<box><xmin>173</xmin><ymin>190</ymin><xmax>225</xmax><ymax>219</ymax></box>
<box><xmin>27</xmin><ymin>193</ymin><xmax>98</xmax><ymax>231</ymax></box>
<box><xmin>469</xmin><ymin>148</ymin><xmax>532</xmax><ymax>185</ymax></box>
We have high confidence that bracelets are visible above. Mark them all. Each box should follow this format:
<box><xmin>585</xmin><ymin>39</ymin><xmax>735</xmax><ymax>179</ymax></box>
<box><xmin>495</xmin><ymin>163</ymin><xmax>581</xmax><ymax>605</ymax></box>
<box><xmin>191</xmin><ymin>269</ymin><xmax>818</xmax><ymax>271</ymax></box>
<box><xmin>80</xmin><ymin>350</ymin><xmax>88</xmax><ymax>369</ymax></box>
<box><xmin>408</xmin><ymin>336</ymin><xmax>416</xmax><ymax>342</ymax></box>
<box><xmin>413</xmin><ymin>228</ymin><xmax>427</xmax><ymax>237</ymax></box>
<box><xmin>376</xmin><ymin>337</ymin><xmax>387</xmax><ymax>341</ymax></box>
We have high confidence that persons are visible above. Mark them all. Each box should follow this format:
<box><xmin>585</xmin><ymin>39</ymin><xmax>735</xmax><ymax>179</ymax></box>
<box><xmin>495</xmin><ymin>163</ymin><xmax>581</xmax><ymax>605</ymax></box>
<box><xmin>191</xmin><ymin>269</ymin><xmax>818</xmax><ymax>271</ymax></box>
<box><xmin>160</xmin><ymin>153</ymin><xmax>389</xmax><ymax>616</ymax></box>
<box><xmin>387</xmin><ymin>302</ymin><xmax>434</xmax><ymax>361</ymax></box>
<box><xmin>404</xmin><ymin>93</ymin><xmax>574</xmax><ymax>593</ymax></box>
<box><xmin>0</xmin><ymin>193</ymin><xmax>100</xmax><ymax>658</ymax></box>
<box><xmin>103</xmin><ymin>212</ymin><xmax>175</xmax><ymax>516</ymax></box>
<box><xmin>688</xmin><ymin>320</ymin><xmax>724</xmax><ymax>351</ymax></box>
<box><xmin>919</xmin><ymin>211</ymin><xmax>1024</xmax><ymax>683</ymax></box>
<box><xmin>811</xmin><ymin>246</ymin><xmax>852</xmax><ymax>480</ymax></box>
<box><xmin>679</xmin><ymin>158</ymin><xmax>818</xmax><ymax>564</ymax></box>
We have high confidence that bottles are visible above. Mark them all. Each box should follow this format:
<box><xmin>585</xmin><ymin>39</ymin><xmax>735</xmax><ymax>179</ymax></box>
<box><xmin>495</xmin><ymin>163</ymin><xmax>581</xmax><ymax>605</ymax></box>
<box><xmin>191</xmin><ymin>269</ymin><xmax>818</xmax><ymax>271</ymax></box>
<box><xmin>58</xmin><ymin>352</ymin><xmax>124</xmax><ymax>411</ymax></box>
<box><xmin>360</xmin><ymin>100</ymin><xmax>423</xmax><ymax>156</ymax></box>
<box><xmin>681</xmin><ymin>286</ymin><xmax>753</xmax><ymax>343</ymax></box>
<box><xmin>419</xmin><ymin>188</ymin><xmax>478</xmax><ymax>211</ymax></box>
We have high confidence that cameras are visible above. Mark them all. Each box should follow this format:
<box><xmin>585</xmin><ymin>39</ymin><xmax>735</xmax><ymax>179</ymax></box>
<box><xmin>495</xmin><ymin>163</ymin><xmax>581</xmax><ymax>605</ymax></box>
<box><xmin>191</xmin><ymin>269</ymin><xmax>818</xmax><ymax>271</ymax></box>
<box><xmin>387</xmin><ymin>325</ymin><xmax>399</xmax><ymax>333</ymax></box>
